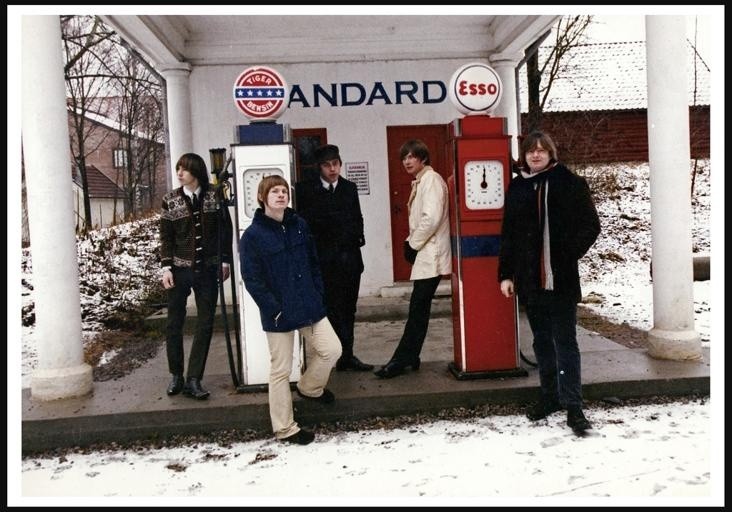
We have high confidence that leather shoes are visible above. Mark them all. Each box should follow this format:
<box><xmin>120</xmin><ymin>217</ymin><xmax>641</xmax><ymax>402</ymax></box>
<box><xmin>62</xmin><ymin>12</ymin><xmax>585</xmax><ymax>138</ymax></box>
<box><xmin>336</xmin><ymin>356</ymin><xmax>374</xmax><ymax>371</ymax></box>
<box><xmin>183</xmin><ymin>376</ymin><xmax>210</xmax><ymax>400</ymax></box>
<box><xmin>167</xmin><ymin>375</ymin><xmax>184</xmax><ymax>395</ymax></box>
<box><xmin>374</xmin><ymin>353</ymin><xmax>420</xmax><ymax>378</ymax></box>
<box><xmin>296</xmin><ymin>386</ymin><xmax>334</xmax><ymax>403</ymax></box>
<box><xmin>567</xmin><ymin>404</ymin><xmax>592</xmax><ymax>432</ymax></box>
<box><xmin>527</xmin><ymin>394</ymin><xmax>563</xmax><ymax>420</ymax></box>
<box><xmin>281</xmin><ymin>429</ymin><xmax>314</xmax><ymax>444</ymax></box>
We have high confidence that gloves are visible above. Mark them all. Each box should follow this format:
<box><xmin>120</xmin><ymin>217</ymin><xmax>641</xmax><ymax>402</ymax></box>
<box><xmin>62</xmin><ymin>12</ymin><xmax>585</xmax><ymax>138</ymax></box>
<box><xmin>404</xmin><ymin>241</ymin><xmax>418</xmax><ymax>264</ymax></box>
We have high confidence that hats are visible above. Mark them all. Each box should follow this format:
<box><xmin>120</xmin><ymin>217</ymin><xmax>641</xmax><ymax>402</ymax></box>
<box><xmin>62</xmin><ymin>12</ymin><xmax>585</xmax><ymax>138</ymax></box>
<box><xmin>314</xmin><ymin>144</ymin><xmax>339</xmax><ymax>163</ymax></box>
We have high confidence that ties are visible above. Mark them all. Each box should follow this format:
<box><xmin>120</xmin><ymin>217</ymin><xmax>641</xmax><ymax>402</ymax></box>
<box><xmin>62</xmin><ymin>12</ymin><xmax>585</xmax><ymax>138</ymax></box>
<box><xmin>193</xmin><ymin>193</ymin><xmax>198</xmax><ymax>209</ymax></box>
<box><xmin>329</xmin><ymin>184</ymin><xmax>333</xmax><ymax>193</ymax></box>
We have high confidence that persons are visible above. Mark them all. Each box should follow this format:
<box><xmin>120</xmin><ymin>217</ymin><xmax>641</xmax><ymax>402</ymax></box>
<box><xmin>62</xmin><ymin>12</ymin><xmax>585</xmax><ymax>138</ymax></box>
<box><xmin>238</xmin><ymin>174</ymin><xmax>343</xmax><ymax>444</ymax></box>
<box><xmin>298</xmin><ymin>144</ymin><xmax>373</xmax><ymax>371</ymax></box>
<box><xmin>497</xmin><ymin>130</ymin><xmax>601</xmax><ymax>434</ymax></box>
<box><xmin>374</xmin><ymin>137</ymin><xmax>452</xmax><ymax>378</ymax></box>
<box><xmin>158</xmin><ymin>152</ymin><xmax>233</xmax><ymax>398</ymax></box>
